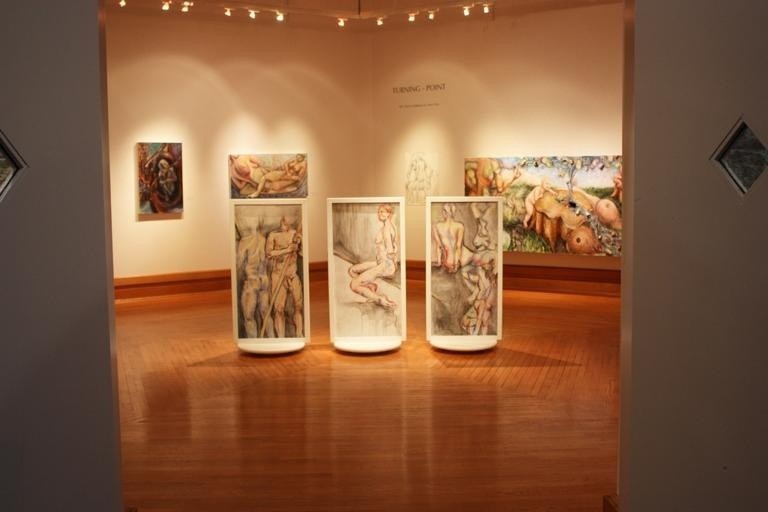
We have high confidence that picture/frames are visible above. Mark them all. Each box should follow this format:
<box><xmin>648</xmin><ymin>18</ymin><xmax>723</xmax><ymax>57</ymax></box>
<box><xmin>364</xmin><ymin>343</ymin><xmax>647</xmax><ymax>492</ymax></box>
<box><xmin>424</xmin><ymin>196</ymin><xmax>503</xmax><ymax>342</ymax></box>
<box><xmin>326</xmin><ymin>196</ymin><xmax>408</xmax><ymax>345</ymax></box>
<box><xmin>227</xmin><ymin>198</ymin><xmax>312</xmax><ymax>346</ymax></box>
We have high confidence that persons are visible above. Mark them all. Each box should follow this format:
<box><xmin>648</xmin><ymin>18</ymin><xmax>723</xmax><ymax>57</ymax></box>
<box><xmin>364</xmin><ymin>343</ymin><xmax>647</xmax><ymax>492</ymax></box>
<box><xmin>236</xmin><ymin>223</ymin><xmax>276</xmax><ymax>338</ymax></box>
<box><xmin>348</xmin><ymin>204</ymin><xmax>398</xmax><ymax>308</ymax></box>
<box><xmin>230</xmin><ymin>154</ymin><xmax>306</xmax><ymax>198</ymax></box>
<box><xmin>431</xmin><ymin>202</ymin><xmax>498</xmax><ymax>335</ymax></box>
<box><xmin>265</xmin><ymin>216</ymin><xmax>302</xmax><ymax>338</ymax></box>
<box><xmin>521</xmin><ymin>177</ymin><xmax>558</xmax><ymax>226</ymax></box>
